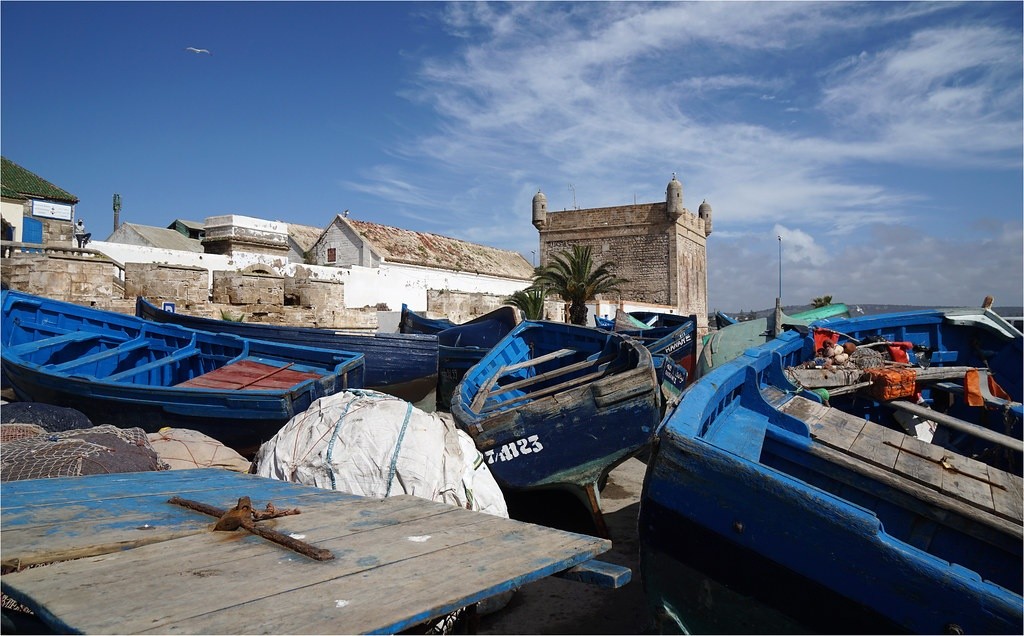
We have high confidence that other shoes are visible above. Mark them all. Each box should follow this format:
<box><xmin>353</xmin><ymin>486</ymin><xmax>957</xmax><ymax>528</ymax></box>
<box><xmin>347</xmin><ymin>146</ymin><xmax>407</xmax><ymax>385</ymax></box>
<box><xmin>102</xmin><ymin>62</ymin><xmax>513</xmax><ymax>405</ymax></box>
<box><xmin>84</xmin><ymin>240</ymin><xmax>87</xmax><ymax>243</ymax></box>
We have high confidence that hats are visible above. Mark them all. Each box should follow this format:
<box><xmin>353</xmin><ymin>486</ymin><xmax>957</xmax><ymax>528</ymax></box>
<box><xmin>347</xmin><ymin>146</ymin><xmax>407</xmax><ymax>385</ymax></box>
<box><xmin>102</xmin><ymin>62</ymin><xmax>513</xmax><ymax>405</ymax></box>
<box><xmin>78</xmin><ymin>219</ymin><xmax>83</xmax><ymax>222</ymax></box>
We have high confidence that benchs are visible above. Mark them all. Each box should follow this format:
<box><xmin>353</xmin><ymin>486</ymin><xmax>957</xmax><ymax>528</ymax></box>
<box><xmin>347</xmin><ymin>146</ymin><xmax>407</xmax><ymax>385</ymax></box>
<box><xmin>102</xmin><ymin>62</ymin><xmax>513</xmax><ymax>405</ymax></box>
<box><xmin>765</xmin><ymin>381</ymin><xmax>1024</xmax><ymax>538</ymax></box>
<box><xmin>479</xmin><ymin>349</ymin><xmax>629</xmax><ymax>412</ymax></box>
<box><xmin>8</xmin><ymin>331</ymin><xmax>201</xmax><ymax>382</ymax></box>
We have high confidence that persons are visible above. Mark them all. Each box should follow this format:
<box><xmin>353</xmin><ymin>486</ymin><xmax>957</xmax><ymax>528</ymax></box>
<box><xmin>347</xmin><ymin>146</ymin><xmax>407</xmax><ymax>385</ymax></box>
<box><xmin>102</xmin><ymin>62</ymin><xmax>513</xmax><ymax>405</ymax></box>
<box><xmin>75</xmin><ymin>218</ymin><xmax>91</xmax><ymax>249</ymax></box>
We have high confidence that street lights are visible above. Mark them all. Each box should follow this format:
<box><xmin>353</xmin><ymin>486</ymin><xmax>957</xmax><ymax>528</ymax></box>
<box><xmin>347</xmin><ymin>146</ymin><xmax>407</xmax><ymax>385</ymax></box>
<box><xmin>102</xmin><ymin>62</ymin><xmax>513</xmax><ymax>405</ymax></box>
<box><xmin>778</xmin><ymin>236</ymin><xmax>782</xmax><ymax>307</ymax></box>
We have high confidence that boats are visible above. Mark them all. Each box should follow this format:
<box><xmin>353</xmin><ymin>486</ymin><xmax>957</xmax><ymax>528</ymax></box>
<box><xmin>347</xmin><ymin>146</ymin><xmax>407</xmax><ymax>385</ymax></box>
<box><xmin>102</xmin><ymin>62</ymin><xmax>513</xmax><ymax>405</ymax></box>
<box><xmin>133</xmin><ymin>297</ymin><xmax>438</xmax><ymax>413</ymax></box>
<box><xmin>0</xmin><ymin>289</ymin><xmax>367</xmax><ymax>455</ymax></box>
<box><xmin>400</xmin><ymin>301</ymin><xmax>1023</xmax><ymax>636</ymax></box>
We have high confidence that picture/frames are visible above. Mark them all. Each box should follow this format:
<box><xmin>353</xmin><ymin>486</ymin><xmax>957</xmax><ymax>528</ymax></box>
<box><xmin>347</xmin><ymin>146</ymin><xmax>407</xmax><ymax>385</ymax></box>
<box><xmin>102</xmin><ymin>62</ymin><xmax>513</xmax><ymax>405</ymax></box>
<box><xmin>32</xmin><ymin>199</ymin><xmax>74</xmax><ymax>222</ymax></box>
